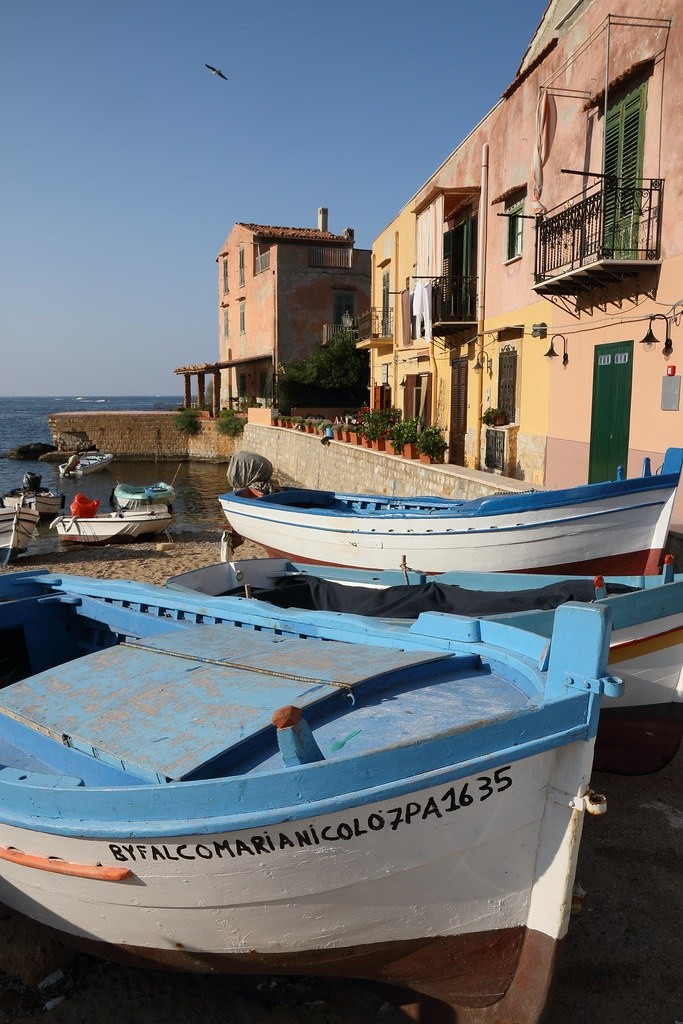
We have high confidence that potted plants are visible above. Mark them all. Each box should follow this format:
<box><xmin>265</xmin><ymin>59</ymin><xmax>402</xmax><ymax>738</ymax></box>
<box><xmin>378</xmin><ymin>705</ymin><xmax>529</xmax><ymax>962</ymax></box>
<box><xmin>389</xmin><ymin>416</ymin><xmax>450</xmax><ymax>464</ymax></box>
<box><xmin>332</xmin><ymin>425</ymin><xmax>361</xmax><ymax>446</ymax></box>
<box><xmin>479</xmin><ymin>407</ymin><xmax>505</xmax><ymax>425</ymax></box>
<box><xmin>364</xmin><ymin>407</ymin><xmax>385</xmax><ymax>451</ymax></box>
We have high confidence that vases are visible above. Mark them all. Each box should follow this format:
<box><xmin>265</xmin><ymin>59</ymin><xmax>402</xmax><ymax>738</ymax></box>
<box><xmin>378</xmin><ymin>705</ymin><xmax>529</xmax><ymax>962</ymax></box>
<box><xmin>361</xmin><ymin>432</ymin><xmax>372</xmax><ymax>448</ymax></box>
<box><xmin>385</xmin><ymin>439</ymin><xmax>400</xmax><ymax>455</ymax></box>
<box><xmin>271</xmin><ymin>419</ymin><xmax>333</xmax><ymax>439</ymax></box>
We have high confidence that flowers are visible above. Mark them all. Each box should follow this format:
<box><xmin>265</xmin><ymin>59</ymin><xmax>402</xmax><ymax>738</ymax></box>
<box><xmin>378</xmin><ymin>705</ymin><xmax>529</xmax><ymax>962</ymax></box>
<box><xmin>381</xmin><ymin>417</ymin><xmax>400</xmax><ymax>439</ymax></box>
<box><xmin>356</xmin><ymin>406</ymin><xmax>373</xmax><ymax>443</ymax></box>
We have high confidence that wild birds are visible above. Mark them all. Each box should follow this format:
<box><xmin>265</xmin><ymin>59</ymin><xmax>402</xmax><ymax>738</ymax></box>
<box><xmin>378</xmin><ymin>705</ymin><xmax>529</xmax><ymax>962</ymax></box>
<box><xmin>204</xmin><ymin>63</ymin><xmax>228</xmax><ymax>81</ymax></box>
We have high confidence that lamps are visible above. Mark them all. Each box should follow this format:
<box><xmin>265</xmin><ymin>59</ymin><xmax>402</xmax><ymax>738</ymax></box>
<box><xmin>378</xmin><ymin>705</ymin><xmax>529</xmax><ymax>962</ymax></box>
<box><xmin>473</xmin><ymin>351</ymin><xmax>492</xmax><ymax>374</ymax></box>
<box><xmin>400</xmin><ymin>374</ymin><xmax>408</xmax><ymax>389</ymax></box>
<box><xmin>544</xmin><ymin>334</ymin><xmax>568</xmax><ymax>367</ymax></box>
<box><xmin>384</xmin><ymin>375</ymin><xmax>393</xmax><ymax>392</ymax></box>
<box><xmin>365</xmin><ymin>377</ymin><xmax>377</xmax><ymax>390</ymax></box>
<box><xmin>639</xmin><ymin>313</ymin><xmax>673</xmax><ymax>352</ymax></box>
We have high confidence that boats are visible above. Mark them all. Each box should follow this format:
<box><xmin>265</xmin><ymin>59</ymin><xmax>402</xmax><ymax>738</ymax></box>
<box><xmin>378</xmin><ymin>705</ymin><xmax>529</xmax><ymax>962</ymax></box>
<box><xmin>164</xmin><ymin>554</ymin><xmax>682</xmax><ymax>711</ymax></box>
<box><xmin>218</xmin><ymin>448</ymin><xmax>683</xmax><ymax>579</ymax></box>
<box><xmin>114</xmin><ymin>481</ymin><xmax>176</xmax><ymax>512</ymax></box>
<box><xmin>2</xmin><ymin>486</ymin><xmax>66</xmax><ymax>522</ymax></box>
<box><xmin>0</xmin><ymin>569</ymin><xmax>624</xmax><ymax>1024</ymax></box>
<box><xmin>0</xmin><ymin>503</ymin><xmax>40</xmax><ymax>565</ymax></box>
<box><xmin>58</xmin><ymin>453</ymin><xmax>114</xmax><ymax>476</ymax></box>
<box><xmin>55</xmin><ymin>504</ymin><xmax>172</xmax><ymax>544</ymax></box>
